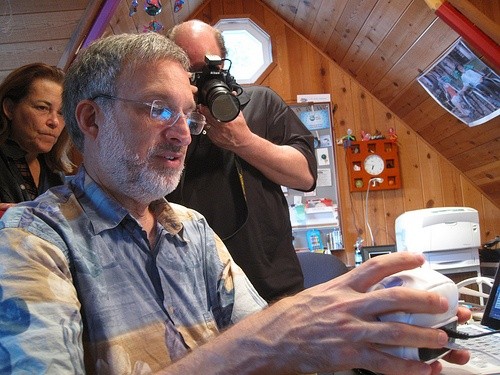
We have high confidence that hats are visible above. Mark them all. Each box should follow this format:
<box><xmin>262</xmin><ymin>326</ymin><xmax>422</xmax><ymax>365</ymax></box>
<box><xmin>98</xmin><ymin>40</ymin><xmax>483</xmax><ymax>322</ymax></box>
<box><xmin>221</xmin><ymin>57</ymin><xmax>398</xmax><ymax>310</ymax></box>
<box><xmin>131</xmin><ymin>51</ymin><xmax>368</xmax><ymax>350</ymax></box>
<box><xmin>454</xmin><ymin>62</ymin><xmax>464</xmax><ymax>70</ymax></box>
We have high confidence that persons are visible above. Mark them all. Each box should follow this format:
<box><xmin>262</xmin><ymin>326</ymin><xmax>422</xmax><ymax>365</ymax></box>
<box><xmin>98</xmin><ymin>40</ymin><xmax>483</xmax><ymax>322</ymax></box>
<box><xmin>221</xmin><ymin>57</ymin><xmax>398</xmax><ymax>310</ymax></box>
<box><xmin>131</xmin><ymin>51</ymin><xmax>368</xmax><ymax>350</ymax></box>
<box><xmin>437</xmin><ymin>62</ymin><xmax>500</xmax><ymax>121</ymax></box>
<box><xmin>0</xmin><ymin>62</ymin><xmax>77</xmax><ymax>219</ymax></box>
<box><xmin>0</xmin><ymin>31</ymin><xmax>472</xmax><ymax>375</ymax></box>
<box><xmin>165</xmin><ymin>20</ymin><xmax>318</xmax><ymax>309</ymax></box>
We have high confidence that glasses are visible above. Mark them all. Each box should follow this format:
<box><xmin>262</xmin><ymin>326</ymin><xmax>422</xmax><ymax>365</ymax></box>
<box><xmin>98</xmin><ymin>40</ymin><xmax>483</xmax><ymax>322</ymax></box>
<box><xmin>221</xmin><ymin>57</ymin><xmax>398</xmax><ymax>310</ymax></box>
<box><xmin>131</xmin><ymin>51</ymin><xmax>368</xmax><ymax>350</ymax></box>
<box><xmin>88</xmin><ymin>94</ymin><xmax>207</xmax><ymax>135</ymax></box>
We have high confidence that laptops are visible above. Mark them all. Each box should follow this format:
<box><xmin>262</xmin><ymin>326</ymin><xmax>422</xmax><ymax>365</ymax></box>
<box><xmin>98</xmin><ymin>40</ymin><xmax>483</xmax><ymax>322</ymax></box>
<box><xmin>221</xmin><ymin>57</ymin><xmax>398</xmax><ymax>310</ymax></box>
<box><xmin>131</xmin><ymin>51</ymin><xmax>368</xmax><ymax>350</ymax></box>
<box><xmin>451</xmin><ymin>263</ymin><xmax>500</xmax><ymax>375</ymax></box>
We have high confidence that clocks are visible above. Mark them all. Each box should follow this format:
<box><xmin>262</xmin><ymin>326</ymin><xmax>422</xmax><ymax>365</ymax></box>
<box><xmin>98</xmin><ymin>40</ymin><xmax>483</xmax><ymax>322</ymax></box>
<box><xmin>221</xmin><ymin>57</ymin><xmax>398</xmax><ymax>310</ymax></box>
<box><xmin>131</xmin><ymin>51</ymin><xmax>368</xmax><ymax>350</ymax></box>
<box><xmin>363</xmin><ymin>154</ymin><xmax>385</xmax><ymax>176</ymax></box>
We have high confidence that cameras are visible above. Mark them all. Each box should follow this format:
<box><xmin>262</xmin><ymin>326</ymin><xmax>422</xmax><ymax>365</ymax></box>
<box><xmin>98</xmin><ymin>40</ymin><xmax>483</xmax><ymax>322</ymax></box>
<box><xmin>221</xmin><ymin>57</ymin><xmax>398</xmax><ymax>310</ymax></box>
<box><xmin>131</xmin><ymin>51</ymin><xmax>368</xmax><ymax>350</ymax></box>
<box><xmin>188</xmin><ymin>55</ymin><xmax>242</xmax><ymax>122</ymax></box>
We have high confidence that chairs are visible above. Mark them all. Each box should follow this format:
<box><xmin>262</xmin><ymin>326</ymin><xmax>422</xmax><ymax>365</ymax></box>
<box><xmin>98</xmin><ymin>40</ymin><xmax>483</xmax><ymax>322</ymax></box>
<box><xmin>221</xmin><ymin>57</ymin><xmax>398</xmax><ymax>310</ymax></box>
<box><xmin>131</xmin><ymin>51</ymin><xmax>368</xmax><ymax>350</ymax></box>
<box><xmin>297</xmin><ymin>252</ymin><xmax>346</xmax><ymax>287</ymax></box>
